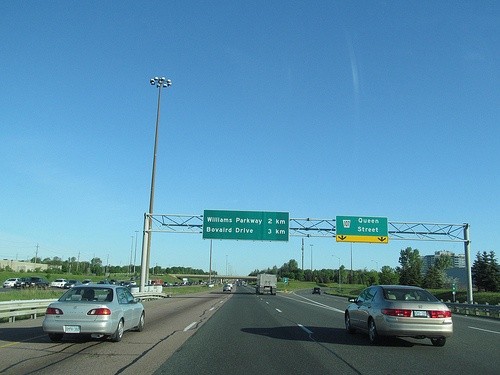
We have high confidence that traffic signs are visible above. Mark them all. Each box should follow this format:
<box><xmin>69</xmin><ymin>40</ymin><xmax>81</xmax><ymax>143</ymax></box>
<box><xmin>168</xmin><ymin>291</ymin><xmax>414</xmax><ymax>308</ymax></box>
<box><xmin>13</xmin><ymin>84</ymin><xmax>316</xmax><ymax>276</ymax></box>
<box><xmin>202</xmin><ymin>210</ymin><xmax>288</xmax><ymax>241</ymax></box>
<box><xmin>335</xmin><ymin>216</ymin><xmax>388</xmax><ymax>243</ymax></box>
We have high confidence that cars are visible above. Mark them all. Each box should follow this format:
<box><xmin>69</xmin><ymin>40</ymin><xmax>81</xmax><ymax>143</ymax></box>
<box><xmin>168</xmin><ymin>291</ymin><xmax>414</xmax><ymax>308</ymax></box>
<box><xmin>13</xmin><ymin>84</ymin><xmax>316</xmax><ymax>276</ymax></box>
<box><xmin>42</xmin><ymin>284</ymin><xmax>146</xmax><ymax>342</ymax></box>
<box><xmin>344</xmin><ymin>284</ymin><xmax>454</xmax><ymax>346</ymax></box>
<box><xmin>3</xmin><ymin>277</ymin><xmax>48</xmax><ymax>289</ymax></box>
<box><xmin>98</xmin><ymin>279</ymin><xmax>137</xmax><ymax>287</ymax></box>
<box><xmin>147</xmin><ymin>280</ymin><xmax>215</xmax><ymax>287</ymax></box>
<box><xmin>312</xmin><ymin>287</ymin><xmax>321</xmax><ymax>295</ymax></box>
<box><xmin>51</xmin><ymin>278</ymin><xmax>92</xmax><ymax>289</ymax></box>
<box><xmin>223</xmin><ymin>283</ymin><xmax>235</xmax><ymax>292</ymax></box>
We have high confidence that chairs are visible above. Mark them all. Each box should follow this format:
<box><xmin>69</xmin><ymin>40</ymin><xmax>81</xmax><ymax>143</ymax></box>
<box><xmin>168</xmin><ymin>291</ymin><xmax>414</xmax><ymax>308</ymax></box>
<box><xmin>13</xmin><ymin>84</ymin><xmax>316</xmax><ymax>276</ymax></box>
<box><xmin>70</xmin><ymin>294</ymin><xmax>82</xmax><ymax>301</ymax></box>
<box><xmin>387</xmin><ymin>294</ymin><xmax>396</xmax><ymax>300</ymax></box>
<box><xmin>97</xmin><ymin>294</ymin><xmax>108</xmax><ymax>301</ymax></box>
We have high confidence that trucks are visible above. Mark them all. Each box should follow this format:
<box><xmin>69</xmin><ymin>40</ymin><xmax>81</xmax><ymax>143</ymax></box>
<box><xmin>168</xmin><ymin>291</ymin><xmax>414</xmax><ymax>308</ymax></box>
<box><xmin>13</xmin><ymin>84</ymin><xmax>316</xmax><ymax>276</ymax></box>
<box><xmin>256</xmin><ymin>274</ymin><xmax>277</xmax><ymax>295</ymax></box>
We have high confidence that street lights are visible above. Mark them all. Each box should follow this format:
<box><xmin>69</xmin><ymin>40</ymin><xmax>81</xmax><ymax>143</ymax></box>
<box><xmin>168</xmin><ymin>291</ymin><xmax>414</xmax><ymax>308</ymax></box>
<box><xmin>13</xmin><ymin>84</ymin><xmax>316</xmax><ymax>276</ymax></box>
<box><xmin>145</xmin><ymin>76</ymin><xmax>173</xmax><ymax>287</ymax></box>
